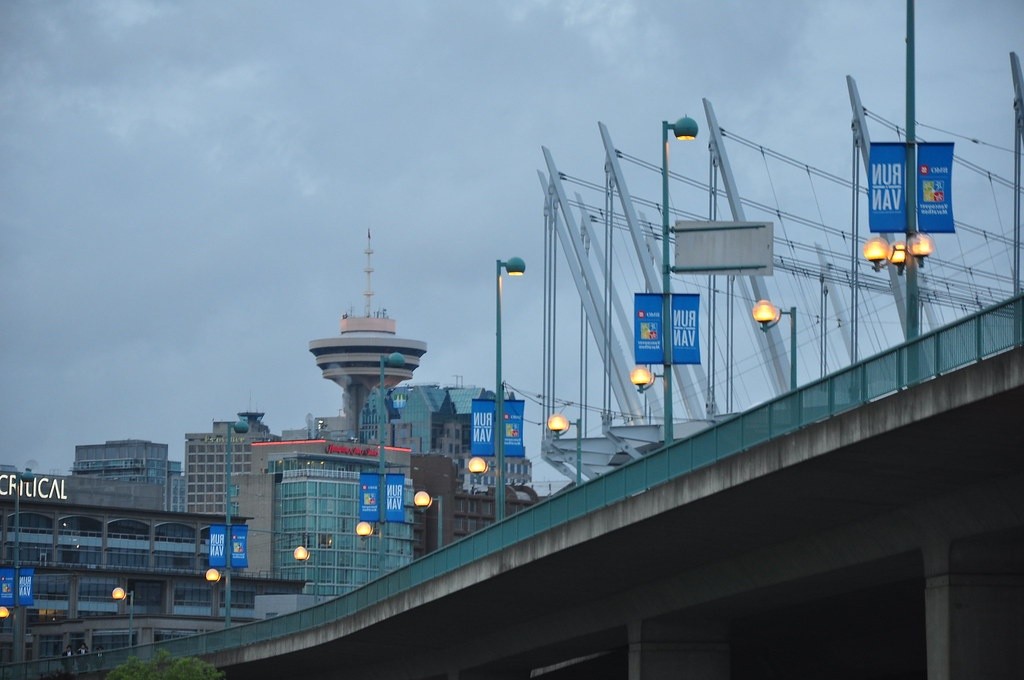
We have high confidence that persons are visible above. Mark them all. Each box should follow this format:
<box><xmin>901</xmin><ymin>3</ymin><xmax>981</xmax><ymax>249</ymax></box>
<box><xmin>60</xmin><ymin>645</ymin><xmax>75</xmax><ymax>674</ymax></box>
<box><xmin>93</xmin><ymin>646</ymin><xmax>106</xmax><ymax>670</ymax></box>
<box><xmin>76</xmin><ymin>643</ymin><xmax>90</xmax><ymax>671</ymax></box>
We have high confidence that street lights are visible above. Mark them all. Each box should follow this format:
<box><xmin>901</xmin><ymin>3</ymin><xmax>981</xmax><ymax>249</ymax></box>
<box><xmin>355</xmin><ymin>349</ymin><xmax>406</xmax><ymax>574</ymax></box>
<box><xmin>859</xmin><ymin>0</ymin><xmax>949</xmax><ymax>347</ymax></box>
<box><xmin>293</xmin><ymin>546</ymin><xmax>323</xmax><ymax>606</ymax></box>
<box><xmin>0</xmin><ymin>466</ymin><xmax>59</xmax><ymax>662</ymax></box>
<box><xmin>546</xmin><ymin>411</ymin><xmax>586</xmax><ymax>487</ymax></box>
<box><xmin>468</xmin><ymin>257</ymin><xmax>526</xmax><ymax>523</ymax></box>
<box><xmin>752</xmin><ymin>300</ymin><xmax>807</xmax><ymax>392</ymax></box>
<box><xmin>111</xmin><ymin>587</ymin><xmax>136</xmax><ymax>646</ymax></box>
<box><xmin>627</xmin><ymin>113</ymin><xmax>704</xmax><ymax>446</ymax></box>
<box><xmin>205</xmin><ymin>420</ymin><xmax>258</xmax><ymax>629</ymax></box>
<box><xmin>414</xmin><ymin>491</ymin><xmax>445</xmax><ymax>549</ymax></box>
